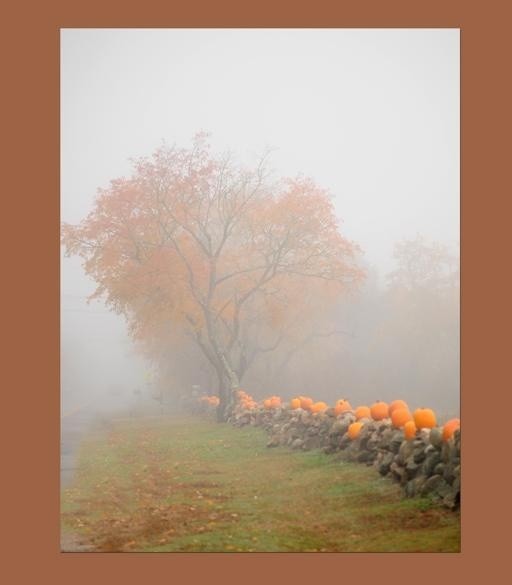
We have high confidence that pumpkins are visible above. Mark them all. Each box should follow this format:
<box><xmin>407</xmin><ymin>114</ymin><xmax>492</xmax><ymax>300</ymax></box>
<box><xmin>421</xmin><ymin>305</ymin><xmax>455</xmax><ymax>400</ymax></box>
<box><xmin>200</xmin><ymin>389</ymin><xmax>460</xmax><ymax>441</ymax></box>
